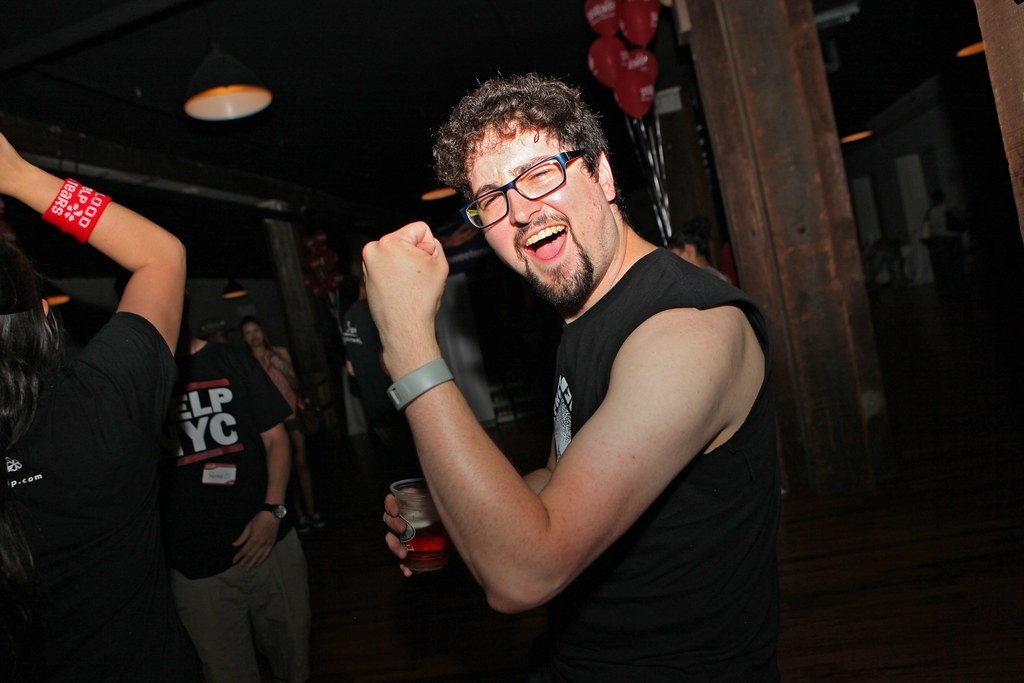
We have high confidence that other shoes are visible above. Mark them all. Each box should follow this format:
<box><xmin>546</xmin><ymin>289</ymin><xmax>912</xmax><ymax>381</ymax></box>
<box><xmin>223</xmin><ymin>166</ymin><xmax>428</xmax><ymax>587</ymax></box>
<box><xmin>298</xmin><ymin>513</ymin><xmax>326</xmax><ymax>533</ymax></box>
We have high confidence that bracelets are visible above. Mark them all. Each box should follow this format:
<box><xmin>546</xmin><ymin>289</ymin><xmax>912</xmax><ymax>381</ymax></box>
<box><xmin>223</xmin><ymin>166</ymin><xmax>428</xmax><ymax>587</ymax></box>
<box><xmin>41</xmin><ymin>176</ymin><xmax>112</xmax><ymax>245</ymax></box>
<box><xmin>387</xmin><ymin>356</ymin><xmax>455</xmax><ymax>410</ymax></box>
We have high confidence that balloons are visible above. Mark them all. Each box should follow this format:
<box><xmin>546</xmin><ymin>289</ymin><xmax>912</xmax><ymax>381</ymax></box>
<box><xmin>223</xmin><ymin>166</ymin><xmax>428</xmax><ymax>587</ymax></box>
<box><xmin>585</xmin><ymin>0</ymin><xmax>660</xmax><ymax>119</ymax></box>
<box><xmin>300</xmin><ymin>230</ymin><xmax>344</xmax><ymax>295</ymax></box>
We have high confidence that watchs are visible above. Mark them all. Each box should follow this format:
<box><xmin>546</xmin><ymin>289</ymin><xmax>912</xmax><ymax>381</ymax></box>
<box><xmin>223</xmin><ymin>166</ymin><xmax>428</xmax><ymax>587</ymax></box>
<box><xmin>258</xmin><ymin>503</ymin><xmax>287</xmax><ymax>519</ymax></box>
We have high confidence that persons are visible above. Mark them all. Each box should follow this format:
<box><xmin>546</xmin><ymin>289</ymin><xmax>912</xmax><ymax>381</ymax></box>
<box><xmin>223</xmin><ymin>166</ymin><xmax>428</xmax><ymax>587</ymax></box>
<box><xmin>668</xmin><ymin>213</ymin><xmax>742</xmax><ymax>288</ymax></box>
<box><xmin>199</xmin><ymin>319</ymin><xmax>232</xmax><ymax>344</ymax></box>
<box><xmin>167</xmin><ymin>328</ymin><xmax>312</xmax><ymax>683</ymax></box>
<box><xmin>240</xmin><ymin>317</ymin><xmax>324</xmax><ymax>533</ymax></box>
<box><xmin>362</xmin><ymin>72</ymin><xmax>785</xmax><ymax>683</ymax></box>
<box><xmin>0</xmin><ymin>132</ymin><xmax>185</xmax><ymax>683</ymax></box>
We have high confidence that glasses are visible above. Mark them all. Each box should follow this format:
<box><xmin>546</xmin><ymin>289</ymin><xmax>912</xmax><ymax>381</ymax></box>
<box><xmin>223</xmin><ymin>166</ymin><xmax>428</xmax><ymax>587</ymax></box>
<box><xmin>461</xmin><ymin>149</ymin><xmax>587</xmax><ymax>229</ymax></box>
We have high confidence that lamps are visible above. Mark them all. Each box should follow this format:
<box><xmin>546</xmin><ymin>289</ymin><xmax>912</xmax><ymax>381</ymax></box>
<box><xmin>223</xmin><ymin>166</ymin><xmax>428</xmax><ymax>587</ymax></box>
<box><xmin>183</xmin><ymin>82</ymin><xmax>275</xmax><ymax>122</ymax></box>
<box><xmin>43</xmin><ymin>282</ymin><xmax>71</xmax><ymax>305</ymax></box>
<box><xmin>220</xmin><ymin>279</ymin><xmax>247</xmax><ymax>300</ymax></box>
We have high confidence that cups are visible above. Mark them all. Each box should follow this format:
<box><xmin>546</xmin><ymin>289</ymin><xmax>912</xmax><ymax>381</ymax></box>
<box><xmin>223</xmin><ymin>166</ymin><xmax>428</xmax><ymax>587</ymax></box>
<box><xmin>388</xmin><ymin>478</ymin><xmax>450</xmax><ymax>573</ymax></box>
